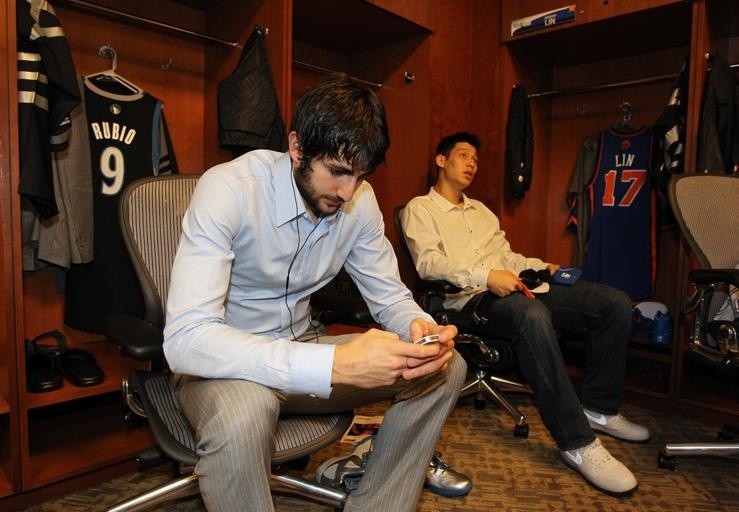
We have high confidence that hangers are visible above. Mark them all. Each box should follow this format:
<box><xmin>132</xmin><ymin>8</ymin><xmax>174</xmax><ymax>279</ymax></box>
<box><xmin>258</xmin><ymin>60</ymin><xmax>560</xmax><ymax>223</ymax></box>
<box><xmin>580</xmin><ymin>101</ymin><xmax>655</xmax><ymax>150</ymax></box>
<box><xmin>81</xmin><ymin>47</ymin><xmax>164</xmax><ymax>112</ymax></box>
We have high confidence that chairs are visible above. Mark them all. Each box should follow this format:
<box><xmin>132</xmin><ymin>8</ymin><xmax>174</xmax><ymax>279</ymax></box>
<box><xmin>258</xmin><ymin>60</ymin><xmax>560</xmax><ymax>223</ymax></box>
<box><xmin>659</xmin><ymin>173</ymin><xmax>738</xmax><ymax>470</ymax></box>
<box><xmin>107</xmin><ymin>175</ymin><xmax>355</xmax><ymax>511</ymax></box>
<box><xmin>395</xmin><ymin>204</ymin><xmax>534</xmax><ymax>438</ymax></box>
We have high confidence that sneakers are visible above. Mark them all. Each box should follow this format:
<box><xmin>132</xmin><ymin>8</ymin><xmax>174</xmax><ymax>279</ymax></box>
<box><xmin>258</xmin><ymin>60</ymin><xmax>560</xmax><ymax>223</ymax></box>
<box><xmin>314</xmin><ymin>434</ymin><xmax>376</xmax><ymax>492</ymax></box>
<box><xmin>424</xmin><ymin>452</ymin><xmax>472</xmax><ymax>498</ymax></box>
<box><xmin>557</xmin><ymin>434</ymin><xmax>640</xmax><ymax>496</ymax></box>
<box><xmin>26</xmin><ymin>334</ymin><xmax>106</xmax><ymax>394</ymax></box>
<box><xmin>582</xmin><ymin>405</ymin><xmax>650</xmax><ymax>444</ymax></box>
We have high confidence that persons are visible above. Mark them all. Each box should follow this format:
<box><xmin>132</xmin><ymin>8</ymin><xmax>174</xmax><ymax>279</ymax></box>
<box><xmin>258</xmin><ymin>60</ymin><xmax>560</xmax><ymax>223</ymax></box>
<box><xmin>162</xmin><ymin>76</ymin><xmax>468</xmax><ymax>512</ymax></box>
<box><xmin>400</xmin><ymin>131</ymin><xmax>651</xmax><ymax>497</ymax></box>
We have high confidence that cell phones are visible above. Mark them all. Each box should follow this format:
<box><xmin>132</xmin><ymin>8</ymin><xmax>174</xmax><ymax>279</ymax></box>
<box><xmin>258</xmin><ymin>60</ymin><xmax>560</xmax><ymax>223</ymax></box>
<box><xmin>414</xmin><ymin>334</ymin><xmax>438</xmax><ymax>344</ymax></box>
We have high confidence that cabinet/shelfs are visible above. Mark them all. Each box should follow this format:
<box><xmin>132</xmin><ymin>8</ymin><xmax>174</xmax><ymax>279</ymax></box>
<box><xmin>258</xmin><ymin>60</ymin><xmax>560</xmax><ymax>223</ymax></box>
<box><xmin>673</xmin><ymin>1</ymin><xmax>739</xmax><ymax>429</ymax></box>
<box><xmin>0</xmin><ymin>0</ymin><xmax>293</xmax><ymax>512</ymax></box>
<box><xmin>502</xmin><ymin>1</ymin><xmax>698</xmax><ymax>425</ymax></box>
<box><xmin>288</xmin><ymin>0</ymin><xmax>435</xmax><ymax>335</ymax></box>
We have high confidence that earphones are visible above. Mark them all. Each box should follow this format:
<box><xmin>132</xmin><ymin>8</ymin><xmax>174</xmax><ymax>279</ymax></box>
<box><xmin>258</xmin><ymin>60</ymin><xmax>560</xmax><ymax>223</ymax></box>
<box><xmin>293</xmin><ymin>142</ymin><xmax>299</xmax><ymax>149</ymax></box>
